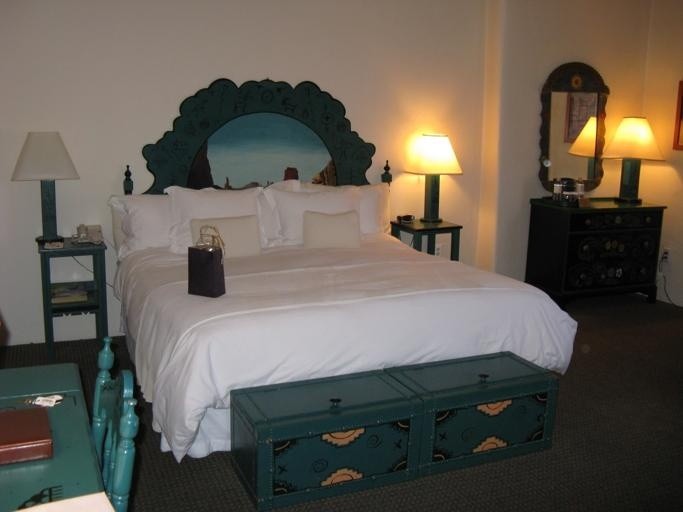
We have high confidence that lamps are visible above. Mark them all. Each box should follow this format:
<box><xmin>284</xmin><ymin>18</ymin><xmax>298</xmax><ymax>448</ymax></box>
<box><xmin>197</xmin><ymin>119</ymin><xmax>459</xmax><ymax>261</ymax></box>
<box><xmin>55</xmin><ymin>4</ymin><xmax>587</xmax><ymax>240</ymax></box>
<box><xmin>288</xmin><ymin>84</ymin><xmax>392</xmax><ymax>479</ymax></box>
<box><xmin>601</xmin><ymin>117</ymin><xmax>666</xmax><ymax>204</ymax></box>
<box><xmin>402</xmin><ymin>135</ymin><xmax>462</xmax><ymax>223</ymax></box>
<box><xmin>568</xmin><ymin>116</ymin><xmax>597</xmax><ymax>159</ymax></box>
<box><xmin>10</xmin><ymin>132</ymin><xmax>79</xmax><ymax>246</ymax></box>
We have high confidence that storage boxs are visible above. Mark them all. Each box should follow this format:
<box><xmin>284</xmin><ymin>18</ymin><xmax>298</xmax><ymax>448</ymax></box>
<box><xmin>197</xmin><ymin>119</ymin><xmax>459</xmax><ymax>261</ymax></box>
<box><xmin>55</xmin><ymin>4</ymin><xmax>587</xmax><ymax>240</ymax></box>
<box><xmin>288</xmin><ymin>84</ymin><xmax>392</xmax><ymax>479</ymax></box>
<box><xmin>383</xmin><ymin>351</ymin><xmax>559</xmax><ymax>478</ymax></box>
<box><xmin>230</xmin><ymin>370</ymin><xmax>424</xmax><ymax>512</ymax></box>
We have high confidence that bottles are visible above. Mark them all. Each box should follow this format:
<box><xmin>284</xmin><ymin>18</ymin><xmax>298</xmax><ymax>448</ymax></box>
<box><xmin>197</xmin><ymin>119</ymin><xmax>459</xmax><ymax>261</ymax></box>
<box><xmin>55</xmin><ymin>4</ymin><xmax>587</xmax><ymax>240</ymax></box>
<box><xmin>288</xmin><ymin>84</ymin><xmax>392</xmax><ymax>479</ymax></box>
<box><xmin>551</xmin><ymin>175</ymin><xmax>583</xmax><ymax>201</ymax></box>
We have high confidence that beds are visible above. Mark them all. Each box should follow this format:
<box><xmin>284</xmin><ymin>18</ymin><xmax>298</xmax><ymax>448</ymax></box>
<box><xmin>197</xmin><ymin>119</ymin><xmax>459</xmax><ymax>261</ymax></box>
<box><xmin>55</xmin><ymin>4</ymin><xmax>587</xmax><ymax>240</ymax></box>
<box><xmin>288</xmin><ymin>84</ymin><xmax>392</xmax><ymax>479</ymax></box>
<box><xmin>113</xmin><ymin>78</ymin><xmax>558</xmax><ymax>463</ymax></box>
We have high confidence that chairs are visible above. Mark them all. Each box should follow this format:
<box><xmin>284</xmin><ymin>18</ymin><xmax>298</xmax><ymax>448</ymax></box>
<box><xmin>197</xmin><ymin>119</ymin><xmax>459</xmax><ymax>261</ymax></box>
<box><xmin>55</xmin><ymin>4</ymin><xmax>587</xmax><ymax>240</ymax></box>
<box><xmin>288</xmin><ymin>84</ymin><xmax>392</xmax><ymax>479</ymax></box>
<box><xmin>90</xmin><ymin>337</ymin><xmax>140</xmax><ymax>512</ymax></box>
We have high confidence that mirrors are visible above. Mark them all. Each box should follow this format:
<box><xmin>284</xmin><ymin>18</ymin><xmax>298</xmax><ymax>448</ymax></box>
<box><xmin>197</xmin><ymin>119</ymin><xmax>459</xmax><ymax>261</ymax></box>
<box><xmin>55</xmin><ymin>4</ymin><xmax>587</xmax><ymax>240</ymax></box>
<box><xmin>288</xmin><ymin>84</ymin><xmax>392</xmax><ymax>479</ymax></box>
<box><xmin>540</xmin><ymin>62</ymin><xmax>610</xmax><ymax>196</ymax></box>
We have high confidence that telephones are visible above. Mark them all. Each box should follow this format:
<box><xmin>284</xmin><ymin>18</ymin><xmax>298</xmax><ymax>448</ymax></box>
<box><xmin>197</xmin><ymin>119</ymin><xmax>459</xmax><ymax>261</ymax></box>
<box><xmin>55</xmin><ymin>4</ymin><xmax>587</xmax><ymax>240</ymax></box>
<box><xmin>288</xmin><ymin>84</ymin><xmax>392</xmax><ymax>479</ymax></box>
<box><xmin>77</xmin><ymin>224</ymin><xmax>102</xmax><ymax>242</ymax></box>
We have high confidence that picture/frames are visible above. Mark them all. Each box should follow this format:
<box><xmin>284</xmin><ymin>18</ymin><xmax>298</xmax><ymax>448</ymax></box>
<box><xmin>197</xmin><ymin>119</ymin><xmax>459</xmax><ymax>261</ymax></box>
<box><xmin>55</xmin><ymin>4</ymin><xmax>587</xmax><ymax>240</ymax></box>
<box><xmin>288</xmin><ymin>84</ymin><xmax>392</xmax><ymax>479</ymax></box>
<box><xmin>672</xmin><ymin>80</ymin><xmax>683</xmax><ymax>150</ymax></box>
<box><xmin>563</xmin><ymin>93</ymin><xmax>598</xmax><ymax>143</ymax></box>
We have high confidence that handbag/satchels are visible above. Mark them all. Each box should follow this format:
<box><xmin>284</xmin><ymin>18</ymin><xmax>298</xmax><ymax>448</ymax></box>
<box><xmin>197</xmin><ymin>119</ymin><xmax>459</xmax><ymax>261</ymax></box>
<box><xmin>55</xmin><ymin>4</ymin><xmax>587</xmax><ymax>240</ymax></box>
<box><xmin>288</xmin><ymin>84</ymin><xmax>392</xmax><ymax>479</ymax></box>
<box><xmin>188</xmin><ymin>225</ymin><xmax>225</xmax><ymax>298</ymax></box>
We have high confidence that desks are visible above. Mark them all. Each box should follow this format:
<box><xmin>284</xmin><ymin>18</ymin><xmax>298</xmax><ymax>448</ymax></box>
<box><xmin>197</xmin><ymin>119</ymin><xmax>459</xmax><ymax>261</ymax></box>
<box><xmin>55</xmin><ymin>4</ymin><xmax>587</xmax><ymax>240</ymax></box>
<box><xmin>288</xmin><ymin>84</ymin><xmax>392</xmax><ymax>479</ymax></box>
<box><xmin>0</xmin><ymin>363</ymin><xmax>105</xmax><ymax>511</ymax></box>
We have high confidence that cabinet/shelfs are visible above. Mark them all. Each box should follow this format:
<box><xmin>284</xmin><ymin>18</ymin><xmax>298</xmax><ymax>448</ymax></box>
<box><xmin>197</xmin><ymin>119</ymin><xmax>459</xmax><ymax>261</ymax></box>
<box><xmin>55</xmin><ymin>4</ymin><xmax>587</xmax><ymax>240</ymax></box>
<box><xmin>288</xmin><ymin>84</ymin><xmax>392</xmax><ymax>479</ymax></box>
<box><xmin>37</xmin><ymin>238</ymin><xmax>108</xmax><ymax>362</ymax></box>
<box><xmin>390</xmin><ymin>219</ymin><xmax>463</xmax><ymax>260</ymax></box>
<box><xmin>524</xmin><ymin>198</ymin><xmax>667</xmax><ymax>312</ymax></box>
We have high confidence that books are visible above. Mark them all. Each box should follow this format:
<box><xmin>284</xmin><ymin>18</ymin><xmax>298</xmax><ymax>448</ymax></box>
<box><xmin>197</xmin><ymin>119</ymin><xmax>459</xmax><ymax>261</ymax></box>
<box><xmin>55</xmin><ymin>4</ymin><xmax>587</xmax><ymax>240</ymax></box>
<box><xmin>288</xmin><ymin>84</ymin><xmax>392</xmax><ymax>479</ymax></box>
<box><xmin>49</xmin><ymin>282</ymin><xmax>87</xmax><ymax>304</ymax></box>
<box><xmin>0</xmin><ymin>405</ymin><xmax>54</xmax><ymax>468</ymax></box>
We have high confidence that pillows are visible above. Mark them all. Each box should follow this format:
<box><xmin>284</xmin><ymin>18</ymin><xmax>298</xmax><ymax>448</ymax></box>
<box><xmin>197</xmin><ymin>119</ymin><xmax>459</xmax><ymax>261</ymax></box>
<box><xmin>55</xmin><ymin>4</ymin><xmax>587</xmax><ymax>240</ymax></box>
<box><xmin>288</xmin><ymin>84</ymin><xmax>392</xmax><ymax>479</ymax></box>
<box><xmin>166</xmin><ymin>185</ymin><xmax>266</xmax><ymax>251</ymax></box>
<box><xmin>267</xmin><ymin>179</ymin><xmax>391</xmax><ymax>246</ymax></box>
<box><xmin>191</xmin><ymin>216</ymin><xmax>261</xmax><ymax>260</ymax></box>
<box><xmin>272</xmin><ymin>187</ymin><xmax>360</xmax><ymax>246</ymax></box>
<box><xmin>120</xmin><ymin>191</ymin><xmax>281</xmax><ymax>260</ymax></box>
<box><xmin>303</xmin><ymin>211</ymin><xmax>361</xmax><ymax>250</ymax></box>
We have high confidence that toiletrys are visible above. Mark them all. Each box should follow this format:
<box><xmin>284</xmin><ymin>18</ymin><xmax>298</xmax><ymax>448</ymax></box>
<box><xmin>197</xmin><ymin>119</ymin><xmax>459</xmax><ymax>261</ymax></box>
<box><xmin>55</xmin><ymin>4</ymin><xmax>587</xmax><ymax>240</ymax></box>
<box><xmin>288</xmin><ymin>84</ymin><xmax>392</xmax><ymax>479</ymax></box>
<box><xmin>551</xmin><ymin>183</ymin><xmax>562</xmax><ymax>202</ymax></box>
<box><xmin>575</xmin><ymin>183</ymin><xmax>585</xmax><ymax>209</ymax></box>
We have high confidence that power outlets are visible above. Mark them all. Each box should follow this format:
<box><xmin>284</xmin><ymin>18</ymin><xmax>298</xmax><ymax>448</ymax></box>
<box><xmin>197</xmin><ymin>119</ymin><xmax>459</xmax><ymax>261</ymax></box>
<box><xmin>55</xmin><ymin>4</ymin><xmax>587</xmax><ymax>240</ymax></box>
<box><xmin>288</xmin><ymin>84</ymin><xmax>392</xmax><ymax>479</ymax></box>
<box><xmin>662</xmin><ymin>250</ymin><xmax>670</xmax><ymax>261</ymax></box>
